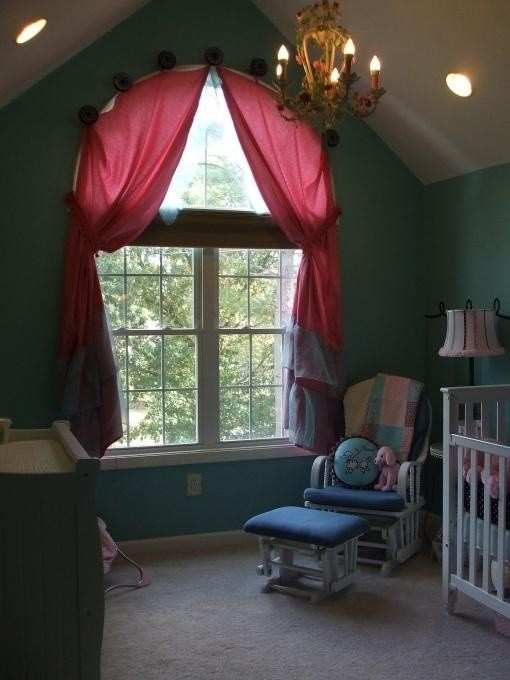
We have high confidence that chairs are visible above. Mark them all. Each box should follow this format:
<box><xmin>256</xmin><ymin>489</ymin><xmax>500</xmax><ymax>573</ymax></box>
<box><xmin>302</xmin><ymin>371</ymin><xmax>432</xmax><ymax>578</ymax></box>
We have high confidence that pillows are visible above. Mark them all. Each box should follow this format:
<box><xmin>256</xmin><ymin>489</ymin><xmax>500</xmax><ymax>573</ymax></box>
<box><xmin>331</xmin><ymin>434</ymin><xmax>383</xmax><ymax>490</ymax></box>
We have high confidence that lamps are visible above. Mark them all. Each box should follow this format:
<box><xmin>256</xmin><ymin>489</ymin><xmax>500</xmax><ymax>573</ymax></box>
<box><xmin>272</xmin><ymin>1</ymin><xmax>384</xmax><ymax>153</ymax></box>
<box><xmin>437</xmin><ymin>306</ymin><xmax>507</xmax><ymax>440</ymax></box>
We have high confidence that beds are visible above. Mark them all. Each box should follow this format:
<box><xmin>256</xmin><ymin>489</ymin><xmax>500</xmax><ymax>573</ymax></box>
<box><xmin>436</xmin><ymin>384</ymin><xmax>509</xmax><ymax>623</ymax></box>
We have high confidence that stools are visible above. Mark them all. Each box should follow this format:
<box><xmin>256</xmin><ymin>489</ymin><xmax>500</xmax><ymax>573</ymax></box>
<box><xmin>245</xmin><ymin>508</ymin><xmax>370</xmax><ymax>606</ymax></box>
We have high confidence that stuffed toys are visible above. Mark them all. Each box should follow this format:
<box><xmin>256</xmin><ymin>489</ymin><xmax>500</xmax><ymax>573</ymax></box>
<box><xmin>463</xmin><ymin>448</ymin><xmax>510</xmax><ymax>498</ymax></box>
<box><xmin>374</xmin><ymin>447</ymin><xmax>400</xmax><ymax>491</ymax></box>
